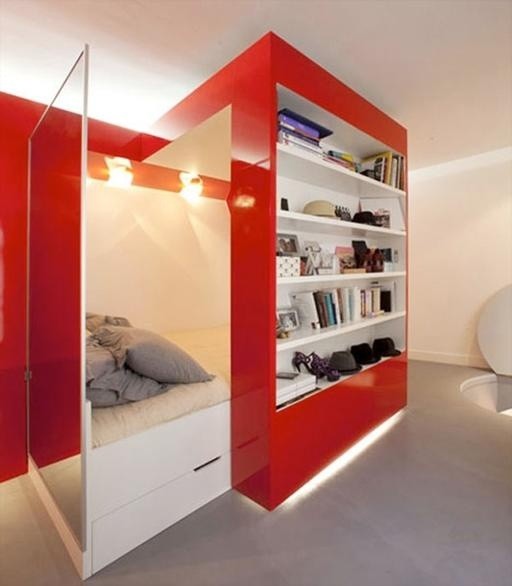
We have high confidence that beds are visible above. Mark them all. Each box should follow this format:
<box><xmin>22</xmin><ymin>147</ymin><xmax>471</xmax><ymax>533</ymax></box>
<box><xmin>91</xmin><ymin>327</ymin><xmax>230</xmax><ymax>451</ymax></box>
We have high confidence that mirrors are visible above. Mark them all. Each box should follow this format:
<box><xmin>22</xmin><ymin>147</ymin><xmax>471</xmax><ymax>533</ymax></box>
<box><xmin>29</xmin><ymin>43</ymin><xmax>84</xmax><ymax>581</ymax></box>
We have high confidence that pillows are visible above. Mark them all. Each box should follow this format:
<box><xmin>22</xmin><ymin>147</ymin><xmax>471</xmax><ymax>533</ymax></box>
<box><xmin>86</xmin><ymin>313</ymin><xmax>130</xmax><ymax>332</ymax></box>
<box><xmin>85</xmin><ymin>335</ymin><xmax>164</xmax><ymax>408</ymax></box>
<box><xmin>88</xmin><ymin>325</ymin><xmax>213</xmax><ymax>384</ymax></box>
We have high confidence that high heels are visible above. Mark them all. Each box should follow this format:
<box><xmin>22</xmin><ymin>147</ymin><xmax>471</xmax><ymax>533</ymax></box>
<box><xmin>311</xmin><ymin>353</ymin><xmax>340</xmax><ymax>384</ymax></box>
<box><xmin>293</xmin><ymin>352</ymin><xmax>326</xmax><ymax>378</ymax></box>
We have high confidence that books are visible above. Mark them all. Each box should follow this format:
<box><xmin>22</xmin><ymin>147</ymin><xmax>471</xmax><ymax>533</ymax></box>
<box><xmin>275</xmin><ymin>373</ymin><xmax>318</xmax><ymax>411</ymax></box>
<box><xmin>290</xmin><ymin>280</ymin><xmax>395</xmax><ymax>329</ymax></box>
<box><xmin>277</xmin><ymin>108</ymin><xmax>405</xmax><ymax>191</ymax></box>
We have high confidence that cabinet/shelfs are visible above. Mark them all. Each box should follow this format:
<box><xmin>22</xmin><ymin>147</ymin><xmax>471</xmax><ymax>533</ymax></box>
<box><xmin>231</xmin><ymin>32</ymin><xmax>408</xmax><ymax>511</ymax></box>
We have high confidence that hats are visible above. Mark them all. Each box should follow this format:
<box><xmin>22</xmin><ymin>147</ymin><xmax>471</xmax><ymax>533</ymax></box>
<box><xmin>328</xmin><ymin>351</ymin><xmax>362</xmax><ymax>374</ymax></box>
<box><xmin>373</xmin><ymin>338</ymin><xmax>401</xmax><ymax>357</ymax></box>
<box><xmin>351</xmin><ymin>343</ymin><xmax>380</xmax><ymax>364</ymax></box>
<box><xmin>352</xmin><ymin>212</ymin><xmax>383</xmax><ymax>226</ymax></box>
<box><xmin>303</xmin><ymin>200</ymin><xmax>336</xmax><ymax>219</ymax></box>
